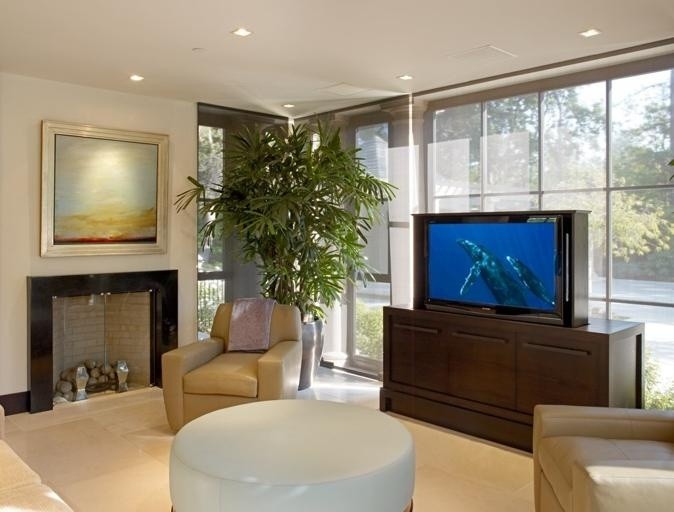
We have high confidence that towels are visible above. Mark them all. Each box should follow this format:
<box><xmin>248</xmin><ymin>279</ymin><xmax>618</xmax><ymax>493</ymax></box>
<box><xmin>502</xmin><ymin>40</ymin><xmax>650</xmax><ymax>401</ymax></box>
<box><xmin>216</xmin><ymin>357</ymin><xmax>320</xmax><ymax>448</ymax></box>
<box><xmin>227</xmin><ymin>297</ymin><xmax>278</xmax><ymax>351</ymax></box>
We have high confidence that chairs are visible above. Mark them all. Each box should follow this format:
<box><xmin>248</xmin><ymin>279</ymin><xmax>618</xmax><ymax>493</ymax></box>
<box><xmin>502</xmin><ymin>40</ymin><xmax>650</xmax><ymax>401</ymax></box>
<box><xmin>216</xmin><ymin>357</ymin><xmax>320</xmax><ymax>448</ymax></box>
<box><xmin>531</xmin><ymin>404</ymin><xmax>674</xmax><ymax>512</ymax></box>
<box><xmin>161</xmin><ymin>302</ymin><xmax>304</xmax><ymax>436</ymax></box>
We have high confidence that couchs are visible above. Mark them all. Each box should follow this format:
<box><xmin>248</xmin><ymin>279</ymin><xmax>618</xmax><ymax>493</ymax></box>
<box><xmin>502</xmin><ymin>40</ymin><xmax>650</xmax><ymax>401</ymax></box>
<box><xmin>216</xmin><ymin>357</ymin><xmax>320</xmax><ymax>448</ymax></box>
<box><xmin>0</xmin><ymin>405</ymin><xmax>75</xmax><ymax>512</ymax></box>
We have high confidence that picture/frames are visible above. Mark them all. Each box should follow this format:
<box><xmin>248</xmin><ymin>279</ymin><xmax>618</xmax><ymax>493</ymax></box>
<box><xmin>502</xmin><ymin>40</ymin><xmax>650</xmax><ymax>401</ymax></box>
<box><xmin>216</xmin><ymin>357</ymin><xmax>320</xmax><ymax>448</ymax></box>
<box><xmin>39</xmin><ymin>119</ymin><xmax>169</xmax><ymax>258</ymax></box>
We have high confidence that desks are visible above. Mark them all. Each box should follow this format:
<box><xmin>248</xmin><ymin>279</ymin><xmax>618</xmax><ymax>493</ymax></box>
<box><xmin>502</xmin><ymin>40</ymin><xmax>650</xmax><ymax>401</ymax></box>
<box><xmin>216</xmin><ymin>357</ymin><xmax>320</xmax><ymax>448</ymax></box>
<box><xmin>169</xmin><ymin>398</ymin><xmax>414</xmax><ymax>512</ymax></box>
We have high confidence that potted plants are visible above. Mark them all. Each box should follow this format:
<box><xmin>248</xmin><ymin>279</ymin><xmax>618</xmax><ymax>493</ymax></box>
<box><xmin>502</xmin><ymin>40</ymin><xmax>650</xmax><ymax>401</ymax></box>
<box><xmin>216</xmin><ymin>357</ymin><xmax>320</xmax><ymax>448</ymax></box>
<box><xmin>173</xmin><ymin>116</ymin><xmax>400</xmax><ymax>392</ymax></box>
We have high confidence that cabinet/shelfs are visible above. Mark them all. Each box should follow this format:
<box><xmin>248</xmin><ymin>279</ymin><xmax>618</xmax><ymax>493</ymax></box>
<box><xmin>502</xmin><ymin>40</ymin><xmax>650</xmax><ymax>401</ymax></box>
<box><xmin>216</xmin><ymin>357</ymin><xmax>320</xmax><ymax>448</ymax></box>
<box><xmin>380</xmin><ymin>307</ymin><xmax>646</xmax><ymax>456</ymax></box>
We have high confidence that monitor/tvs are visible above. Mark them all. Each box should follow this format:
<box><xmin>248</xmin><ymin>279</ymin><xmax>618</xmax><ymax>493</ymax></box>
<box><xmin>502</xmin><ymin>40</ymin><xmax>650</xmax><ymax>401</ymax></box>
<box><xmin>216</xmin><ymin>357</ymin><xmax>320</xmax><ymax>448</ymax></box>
<box><xmin>423</xmin><ymin>215</ymin><xmax>570</xmax><ymax>327</ymax></box>
